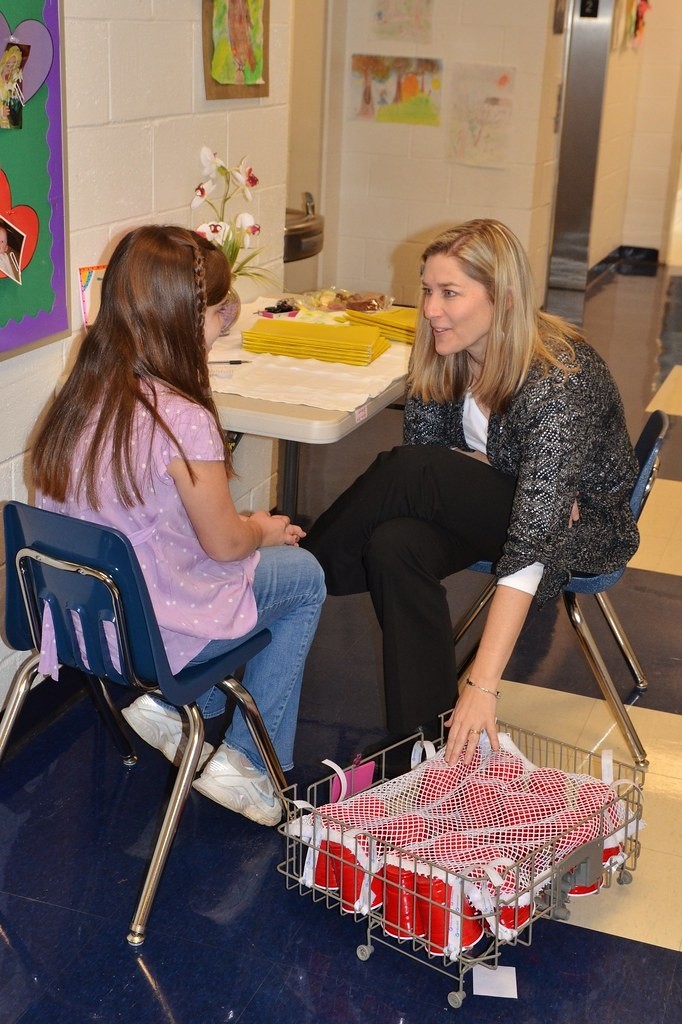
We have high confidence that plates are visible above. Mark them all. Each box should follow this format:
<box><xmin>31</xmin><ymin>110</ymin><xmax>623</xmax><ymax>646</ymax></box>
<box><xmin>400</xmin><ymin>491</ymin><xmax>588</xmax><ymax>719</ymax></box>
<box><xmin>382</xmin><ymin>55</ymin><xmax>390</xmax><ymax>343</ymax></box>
<box><xmin>298</xmin><ymin>285</ymin><xmax>395</xmax><ymax>316</ymax></box>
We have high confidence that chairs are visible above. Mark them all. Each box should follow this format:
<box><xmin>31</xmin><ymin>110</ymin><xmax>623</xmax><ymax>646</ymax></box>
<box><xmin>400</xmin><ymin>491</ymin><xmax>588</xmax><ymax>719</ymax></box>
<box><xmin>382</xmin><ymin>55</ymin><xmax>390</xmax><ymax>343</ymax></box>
<box><xmin>452</xmin><ymin>410</ymin><xmax>670</xmax><ymax>766</ymax></box>
<box><xmin>0</xmin><ymin>499</ymin><xmax>301</xmax><ymax>946</ymax></box>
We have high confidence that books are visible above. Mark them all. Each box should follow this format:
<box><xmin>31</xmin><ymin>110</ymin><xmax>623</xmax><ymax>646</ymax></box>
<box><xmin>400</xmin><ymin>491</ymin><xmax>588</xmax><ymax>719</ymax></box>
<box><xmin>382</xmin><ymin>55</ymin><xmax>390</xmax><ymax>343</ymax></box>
<box><xmin>242</xmin><ymin>308</ymin><xmax>420</xmax><ymax>366</ymax></box>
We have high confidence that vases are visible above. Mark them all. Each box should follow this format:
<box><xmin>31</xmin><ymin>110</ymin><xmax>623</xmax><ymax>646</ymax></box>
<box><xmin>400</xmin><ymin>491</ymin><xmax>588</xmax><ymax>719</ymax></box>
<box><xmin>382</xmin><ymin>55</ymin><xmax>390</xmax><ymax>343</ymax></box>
<box><xmin>217</xmin><ymin>288</ymin><xmax>240</xmax><ymax>336</ymax></box>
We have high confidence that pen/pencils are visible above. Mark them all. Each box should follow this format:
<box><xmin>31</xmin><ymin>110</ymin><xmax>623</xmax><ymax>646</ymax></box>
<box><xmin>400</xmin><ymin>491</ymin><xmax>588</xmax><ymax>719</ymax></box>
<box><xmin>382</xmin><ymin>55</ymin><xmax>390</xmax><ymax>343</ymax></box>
<box><xmin>207</xmin><ymin>359</ymin><xmax>252</xmax><ymax>365</ymax></box>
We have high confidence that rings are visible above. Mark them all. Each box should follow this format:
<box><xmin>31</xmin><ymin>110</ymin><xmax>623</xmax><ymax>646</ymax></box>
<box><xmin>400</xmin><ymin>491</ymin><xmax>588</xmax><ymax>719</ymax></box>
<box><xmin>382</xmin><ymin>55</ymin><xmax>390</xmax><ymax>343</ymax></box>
<box><xmin>470</xmin><ymin>730</ymin><xmax>481</xmax><ymax>734</ymax></box>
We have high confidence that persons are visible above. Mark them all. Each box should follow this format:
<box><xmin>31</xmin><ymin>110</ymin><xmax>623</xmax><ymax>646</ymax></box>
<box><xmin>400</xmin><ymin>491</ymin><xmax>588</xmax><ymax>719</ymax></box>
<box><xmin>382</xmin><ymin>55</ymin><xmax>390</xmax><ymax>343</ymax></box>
<box><xmin>33</xmin><ymin>225</ymin><xmax>327</xmax><ymax>827</ymax></box>
<box><xmin>0</xmin><ymin>227</ymin><xmax>20</xmax><ymax>280</ymax></box>
<box><xmin>299</xmin><ymin>220</ymin><xmax>638</xmax><ymax>779</ymax></box>
<box><xmin>0</xmin><ymin>46</ymin><xmax>22</xmax><ymax>127</ymax></box>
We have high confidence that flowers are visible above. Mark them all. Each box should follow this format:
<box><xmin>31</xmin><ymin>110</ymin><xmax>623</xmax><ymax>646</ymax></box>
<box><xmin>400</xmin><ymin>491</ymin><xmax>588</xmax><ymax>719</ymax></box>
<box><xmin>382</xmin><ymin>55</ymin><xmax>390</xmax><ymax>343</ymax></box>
<box><xmin>189</xmin><ymin>145</ymin><xmax>285</xmax><ymax>293</ymax></box>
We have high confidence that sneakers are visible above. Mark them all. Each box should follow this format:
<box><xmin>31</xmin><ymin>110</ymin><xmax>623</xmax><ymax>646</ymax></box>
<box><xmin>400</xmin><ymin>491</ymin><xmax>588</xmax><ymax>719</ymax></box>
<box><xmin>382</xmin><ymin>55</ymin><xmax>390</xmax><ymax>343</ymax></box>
<box><xmin>190</xmin><ymin>744</ymin><xmax>282</xmax><ymax>826</ymax></box>
<box><xmin>121</xmin><ymin>688</ymin><xmax>216</xmax><ymax>773</ymax></box>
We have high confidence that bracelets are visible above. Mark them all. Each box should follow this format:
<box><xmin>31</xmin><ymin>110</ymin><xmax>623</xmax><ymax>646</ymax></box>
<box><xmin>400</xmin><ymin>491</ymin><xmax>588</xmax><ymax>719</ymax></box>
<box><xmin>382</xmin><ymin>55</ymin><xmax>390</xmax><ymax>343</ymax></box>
<box><xmin>466</xmin><ymin>679</ymin><xmax>500</xmax><ymax>697</ymax></box>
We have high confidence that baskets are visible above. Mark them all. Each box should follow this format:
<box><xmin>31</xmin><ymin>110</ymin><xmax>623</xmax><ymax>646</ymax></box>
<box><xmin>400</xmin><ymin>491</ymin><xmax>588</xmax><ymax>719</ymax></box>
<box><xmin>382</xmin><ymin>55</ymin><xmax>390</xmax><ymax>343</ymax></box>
<box><xmin>277</xmin><ymin>709</ymin><xmax>647</xmax><ymax>989</ymax></box>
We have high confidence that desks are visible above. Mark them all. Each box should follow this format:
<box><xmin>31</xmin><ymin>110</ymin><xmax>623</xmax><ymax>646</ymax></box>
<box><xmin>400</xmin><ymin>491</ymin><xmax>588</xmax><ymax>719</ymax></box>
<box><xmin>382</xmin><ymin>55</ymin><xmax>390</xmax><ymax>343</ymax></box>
<box><xmin>208</xmin><ymin>293</ymin><xmax>412</xmax><ymax>522</ymax></box>
